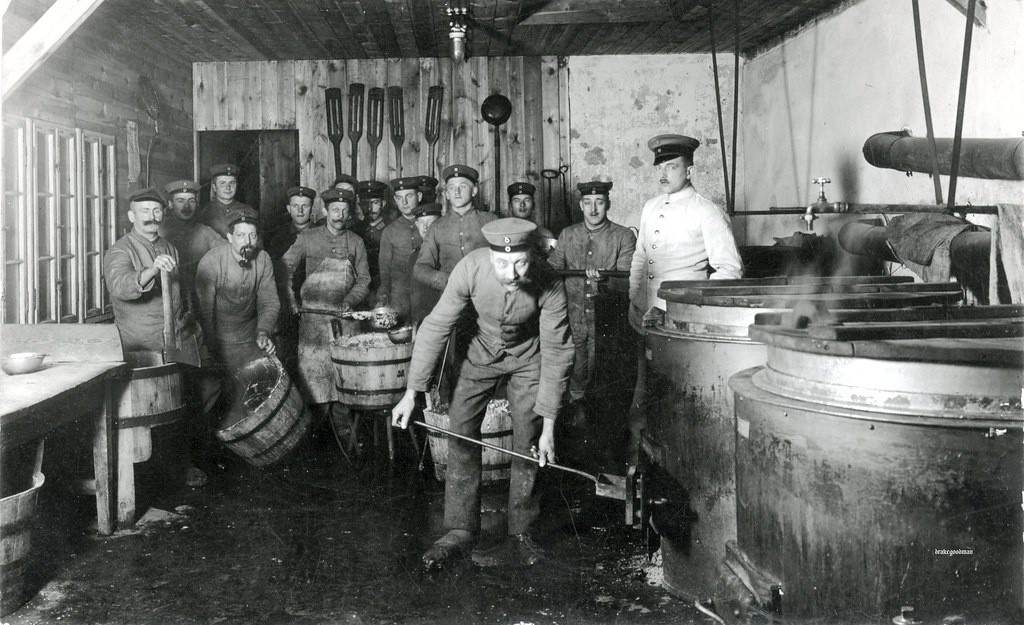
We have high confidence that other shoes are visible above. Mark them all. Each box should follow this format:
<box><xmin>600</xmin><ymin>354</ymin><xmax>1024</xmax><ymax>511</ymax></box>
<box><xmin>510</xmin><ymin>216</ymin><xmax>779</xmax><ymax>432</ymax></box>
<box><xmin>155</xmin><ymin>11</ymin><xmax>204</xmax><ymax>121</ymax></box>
<box><xmin>515</xmin><ymin>532</ymin><xmax>543</xmax><ymax>563</ymax></box>
<box><xmin>179</xmin><ymin>468</ymin><xmax>205</xmax><ymax>488</ymax></box>
<box><xmin>422</xmin><ymin>529</ymin><xmax>479</xmax><ymax>565</ymax></box>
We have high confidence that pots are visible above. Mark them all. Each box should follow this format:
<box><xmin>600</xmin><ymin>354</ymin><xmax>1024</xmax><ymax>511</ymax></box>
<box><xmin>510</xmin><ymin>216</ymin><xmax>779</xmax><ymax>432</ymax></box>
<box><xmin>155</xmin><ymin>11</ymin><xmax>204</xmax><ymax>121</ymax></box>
<box><xmin>387</xmin><ymin>328</ymin><xmax>415</xmax><ymax>344</ymax></box>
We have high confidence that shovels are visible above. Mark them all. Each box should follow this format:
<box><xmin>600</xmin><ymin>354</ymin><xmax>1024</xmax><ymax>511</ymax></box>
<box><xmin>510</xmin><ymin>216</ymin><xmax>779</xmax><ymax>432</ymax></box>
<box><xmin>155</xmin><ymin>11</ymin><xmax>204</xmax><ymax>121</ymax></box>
<box><xmin>424</xmin><ymin>86</ymin><xmax>443</xmax><ymax>178</ymax></box>
<box><xmin>325</xmin><ymin>88</ymin><xmax>343</xmax><ymax>177</ymax></box>
<box><xmin>348</xmin><ymin>83</ymin><xmax>364</xmax><ymax>179</ymax></box>
<box><xmin>388</xmin><ymin>86</ymin><xmax>405</xmax><ymax>178</ymax></box>
<box><xmin>367</xmin><ymin>87</ymin><xmax>384</xmax><ymax>181</ymax></box>
<box><xmin>413</xmin><ymin>421</ymin><xmax>641</xmax><ymax>500</ymax></box>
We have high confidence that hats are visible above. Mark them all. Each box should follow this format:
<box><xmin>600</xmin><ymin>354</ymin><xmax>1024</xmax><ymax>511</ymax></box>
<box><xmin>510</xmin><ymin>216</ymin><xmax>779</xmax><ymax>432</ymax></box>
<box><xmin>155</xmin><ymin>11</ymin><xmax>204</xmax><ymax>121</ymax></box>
<box><xmin>165</xmin><ymin>180</ymin><xmax>201</xmax><ymax>196</ymax></box>
<box><xmin>415</xmin><ymin>175</ymin><xmax>439</xmax><ymax>189</ymax></box>
<box><xmin>390</xmin><ymin>177</ymin><xmax>422</xmax><ymax>191</ymax></box>
<box><xmin>357</xmin><ymin>181</ymin><xmax>387</xmax><ymax>199</ymax></box>
<box><xmin>411</xmin><ymin>203</ymin><xmax>442</xmax><ymax>219</ymax></box>
<box><xmin>225</xmin><ymin>208</ymin><xmax>260</xmax><ymax>227</ymax></box>
<box><xmin>210</xmin><ymin>163</ymin><xmax>240</xmax><ymax>179</ymax></box>
<box><xmin>286</xmin><ymin>186</ymin><xmax>316</xmax><ymax>203</ymax></box>
<box><xmin>321</xmin><ymin>188</ymin><xmax>353</xmax><ymax>204</ymax></box>
<box><xmin>481</xmin><ymin>216</ymin><xmax>537</xmax><ymax>251</ymax></box>
<box><xmin>648</xmin><ymin>134</ymin><xmax>700</xmax><ymax>166</ymax></box>
<box><xmin>126</xmin><ymin>187</ymin><xmax>167</xmax><ymax>207</ymax></box>
<box><xmin>334</xmin><ymin>174</ymin><xmax>358</xmax><ymax>192</ymax></box>
<box><xmin>577</xmin><ymin>181</ymin><xmax>614</xmax><ymax>196</ymax></box>
<box><xmin>443</xmin><ymin>164</ymin><xmax>480</xmax><ymax>185</ymax></box>
<box><xmin>507</xmin><ymin>182</ymin><xmax>536</xmax><ymax>200</ymax></box>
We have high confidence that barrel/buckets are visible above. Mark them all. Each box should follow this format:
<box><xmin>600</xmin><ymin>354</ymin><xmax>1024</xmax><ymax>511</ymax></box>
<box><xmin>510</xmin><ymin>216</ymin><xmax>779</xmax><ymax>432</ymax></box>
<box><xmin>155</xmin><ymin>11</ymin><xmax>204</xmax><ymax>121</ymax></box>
<box><xmin>206</xmin><ymin>348</ymin><xmax>315</xmax><ymax>473</ymax></box>
<box><xmin>114</xmin><ymin>351</ymin><xmax>190</xmax><ymax>427</ymax></box>
<box><xmin>422</xmin><ymin>400</ymin><xmax>518</xmax><ymax>490</ymax></box>
<box><xmin>331</xmin><ymin>332</ymin><xmax>418</xmax><ymax>409</ymax></box>
<box><xmin>1</xmin><ymin>440</ymin><xmax>46</xmax><ymax>618</ymax></box>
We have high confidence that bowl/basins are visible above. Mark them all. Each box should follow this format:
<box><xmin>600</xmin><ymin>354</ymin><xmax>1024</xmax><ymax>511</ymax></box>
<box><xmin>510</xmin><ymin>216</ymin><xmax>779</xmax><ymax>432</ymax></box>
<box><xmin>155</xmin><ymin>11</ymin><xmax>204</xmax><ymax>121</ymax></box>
<box><xmin>0</xmin><ymin>351</ymin><xmax>49</xmax><ymax>374</ymax></box>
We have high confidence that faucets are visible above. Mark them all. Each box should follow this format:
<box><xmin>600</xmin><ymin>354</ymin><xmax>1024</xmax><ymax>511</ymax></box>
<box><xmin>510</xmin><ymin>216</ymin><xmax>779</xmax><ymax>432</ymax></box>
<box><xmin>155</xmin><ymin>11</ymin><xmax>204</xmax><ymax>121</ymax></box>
<box><xmin>804</xmin><ymin>177</ymin><xmax>846</xmax><ymax>231</ymax></box>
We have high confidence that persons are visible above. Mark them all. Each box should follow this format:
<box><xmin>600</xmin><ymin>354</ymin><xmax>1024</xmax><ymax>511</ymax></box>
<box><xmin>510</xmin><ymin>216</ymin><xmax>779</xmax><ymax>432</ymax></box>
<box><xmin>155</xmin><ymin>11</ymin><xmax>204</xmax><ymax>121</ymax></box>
<box><xmin>264</xmin><ymin>173</ymin><xmax>443</xmax><ymax>453</ymax></box>
<box><xmin>106</xmin><ymin>191</ymin><xmax>220</xmax><ymax>487</ymax></box>
<box><xmin>413</xmin><ymin>165</ymin><xmax>509</xmax><ymax>399</ymax></box>
<box><xmin>392</xmin><ymin>217</ymin><xmax>576</xmax><ymax>572</ymax></box>
<box><xmin>547</xmin><ymin>180</ymin><xmax>637</xmax><ymax>433</ymax></box>
<box><xmin>508</xmin><ymin>182</ymin><xmax>552</xmax><ymax>251</ymax></box>
<box><xmin>622</xmin><ymin>136</ymin><xmax>745</xmax><ymax>475</ymax></box>
<box><xmin>165</xmin><ymin>165</ymin><xmax>279</xmax><ymax>449</ymax></box>
